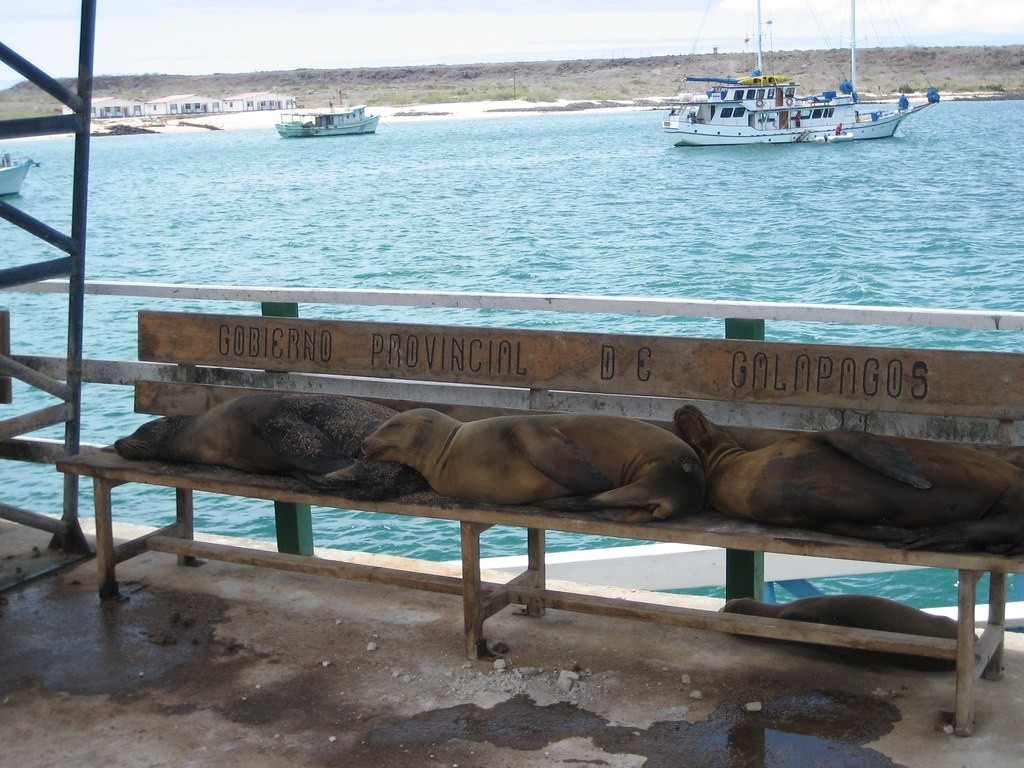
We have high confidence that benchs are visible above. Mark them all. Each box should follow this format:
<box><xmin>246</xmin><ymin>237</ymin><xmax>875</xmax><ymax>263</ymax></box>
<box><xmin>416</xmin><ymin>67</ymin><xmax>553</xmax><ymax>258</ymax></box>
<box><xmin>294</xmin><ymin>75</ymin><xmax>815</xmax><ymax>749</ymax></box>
<box><xmin>56</xmin><ymin>309</ymin><xmax>1024</xmax><ymax>737</ymax></box>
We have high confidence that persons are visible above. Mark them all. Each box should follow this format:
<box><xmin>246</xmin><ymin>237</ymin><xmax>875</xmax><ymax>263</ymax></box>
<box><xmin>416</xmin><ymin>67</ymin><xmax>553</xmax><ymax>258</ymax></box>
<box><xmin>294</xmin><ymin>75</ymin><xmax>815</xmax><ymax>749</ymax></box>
<box><xmin>836</xmin><ymin>123</ymin><xmax>843</xmax><ymax>136</ymax></box>
<box><xmin>855</xmin><ymin>112</ymin><xmax>861</xmax><ymax>122</ymax></box>
<box><xmin>795</xmin><ymin>110</ymin><xmax>801</xmax><ymax>128</ymax></box>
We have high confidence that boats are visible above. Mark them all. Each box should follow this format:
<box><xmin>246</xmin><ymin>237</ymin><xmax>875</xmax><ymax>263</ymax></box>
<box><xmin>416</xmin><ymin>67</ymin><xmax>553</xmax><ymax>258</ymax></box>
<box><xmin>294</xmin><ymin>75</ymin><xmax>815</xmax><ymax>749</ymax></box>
<box><xmin>276</xmin><ymin>102</ymin><xmax>381</xmax><ymax>138</ymax></box>
<box><xmin>663</xmin><ymin>0</ymin><xmax>940</xmax><ymax>146</ymax></box>
<box><xmin>0</xmin><ymin>151</ymin><xmax>41</xmax><ymax>195</ymax></box>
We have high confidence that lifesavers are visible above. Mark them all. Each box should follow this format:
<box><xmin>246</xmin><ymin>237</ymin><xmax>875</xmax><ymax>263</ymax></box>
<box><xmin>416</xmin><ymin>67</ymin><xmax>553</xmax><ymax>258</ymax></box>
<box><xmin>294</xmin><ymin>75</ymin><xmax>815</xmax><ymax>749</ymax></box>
<box><xmin>755</xmin><ymin>99</ymin><xmax>763</xmax><ymax>107</ymax></box>
<box><xmin>786</xmin><ymin>97</ymin><xmax>793</xmax><ymax>105</ymax></box>
